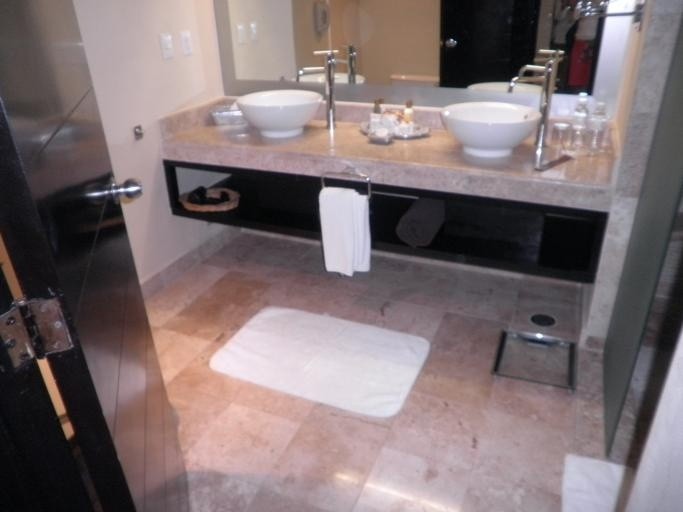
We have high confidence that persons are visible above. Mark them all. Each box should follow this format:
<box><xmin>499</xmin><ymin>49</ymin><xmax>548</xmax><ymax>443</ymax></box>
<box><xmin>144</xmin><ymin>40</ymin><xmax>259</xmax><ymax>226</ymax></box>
<box><xmin>549</xmin><ymin>0</ymin><xmax>607</xmax><ymax>93</ymax></box>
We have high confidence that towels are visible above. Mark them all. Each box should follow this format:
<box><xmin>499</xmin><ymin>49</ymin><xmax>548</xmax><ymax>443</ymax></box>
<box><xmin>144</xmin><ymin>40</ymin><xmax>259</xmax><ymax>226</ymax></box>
<box><xmin>317</xmin><ymin>185</ymin><xmax>372</xmax><ymax>278</ymax></box>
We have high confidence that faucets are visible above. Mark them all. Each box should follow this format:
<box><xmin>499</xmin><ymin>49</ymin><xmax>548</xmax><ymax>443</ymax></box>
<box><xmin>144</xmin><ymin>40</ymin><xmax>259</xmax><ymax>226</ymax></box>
<box><xmin>337</xmin><ymin>45</ymin><xmax>356</xmax><ymax>83</ymax></box>
<box><xmin>509</xmin><ymin>64</ymin><xmax>556</xmax><ymax>156</ymax></box>
<box><xmin>298</xmin><ymin>50</ymin><xmax>336</xmax><ymax>128</ymax></box>
<box><xmin>533</xmin><ymin>48</ymin><xmax>566</xmax><ymax>66</ymax></box>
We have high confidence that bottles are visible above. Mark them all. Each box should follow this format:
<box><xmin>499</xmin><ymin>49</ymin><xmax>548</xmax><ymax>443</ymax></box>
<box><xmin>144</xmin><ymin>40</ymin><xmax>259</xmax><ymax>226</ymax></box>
<box><xmin>553</xmin><ymin>94</ymin><xmax>612</xmax><ymax>154</ymax></box>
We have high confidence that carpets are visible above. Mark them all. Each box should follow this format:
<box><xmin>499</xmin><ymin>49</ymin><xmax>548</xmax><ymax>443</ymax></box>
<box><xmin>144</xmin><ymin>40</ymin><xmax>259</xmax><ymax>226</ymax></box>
<box><xmin>206</xmin><ymin>304</ymin><xmax>431</xmax><ymax>421</ymax></box>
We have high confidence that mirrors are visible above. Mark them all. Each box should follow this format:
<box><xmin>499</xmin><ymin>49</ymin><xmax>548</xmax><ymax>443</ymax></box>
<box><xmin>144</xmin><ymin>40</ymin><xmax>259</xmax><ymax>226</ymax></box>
<box><xmin>212</xmin><ymin>1</ymin><xmax>637</xmax><ymax>120</ymax></box>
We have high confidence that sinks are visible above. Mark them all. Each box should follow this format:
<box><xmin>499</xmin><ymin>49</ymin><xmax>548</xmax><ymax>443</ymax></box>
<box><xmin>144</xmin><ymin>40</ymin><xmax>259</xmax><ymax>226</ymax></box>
<box><xmin>440</xmin><ymin>101</ymin><xmax>541</xmax><ymax>156</ymax></box>
<box><xmin>466</xmin><ymin>82</ymin><xmax>544</xmax><ymax>96</ymax></box>
<box><xmin>294</xmin><ymin>73</ymin><xmax>365</xmax><ymax>86</ymax></box>
<box><xmin>237</xmin><ymin>89</ymin><xmax>323</xmax><ymax>139</ymax></box>
